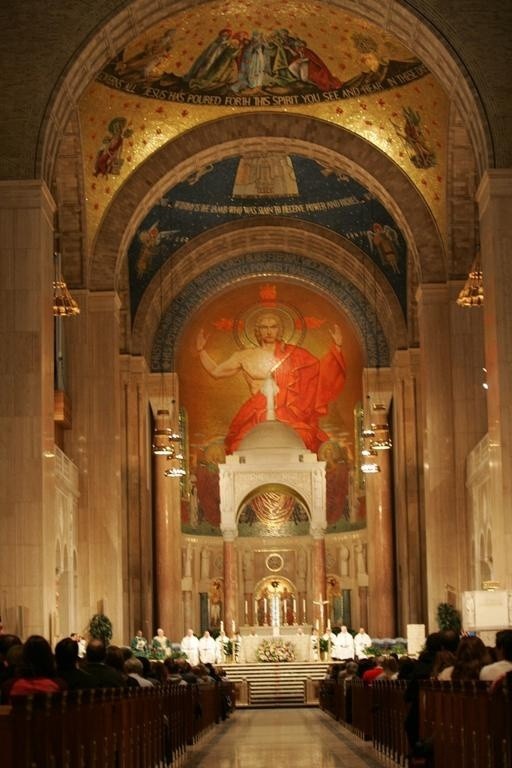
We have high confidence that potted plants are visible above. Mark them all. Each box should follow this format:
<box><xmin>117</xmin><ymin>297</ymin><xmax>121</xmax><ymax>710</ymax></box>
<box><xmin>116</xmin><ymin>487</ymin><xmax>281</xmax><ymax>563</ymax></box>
<box><xmin>88</xmin><ymin>613</ymin><xmax>112</xmax><ymax>649</ymax></box>
<box><xmin>437</xmin><ymin>601</ymin><xmax>461</xmax><ymax>640</ymax></box>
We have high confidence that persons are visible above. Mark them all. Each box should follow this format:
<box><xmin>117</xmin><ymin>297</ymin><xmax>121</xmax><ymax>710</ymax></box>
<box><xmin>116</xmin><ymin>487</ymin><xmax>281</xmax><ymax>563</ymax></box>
<box><xmin>97</xmin><ymin>120</ymin><xmax>132</xmax><ymax>177</ymax></box>
<box><xmin>198</xmin><ymin>630</ymin><xmax>219</xmax><ymax>664</ymax></box>
<box><xmin>122</xmin><ymin>27</ymin><xmax>178</xmax><ymax>72</ymax></box>
<box><xmin>151</xmin><ymin>627</ymin><xmax>170</xmax><ymax>659</ymax></box>
<box><xmin>194</xmin><ymin>309</ymin><xmax>351</xmax><ymax>467</ymax></box>
<box><xmin>216</xmin><ymin>630</ymin><xmax>229</xmax><ymax>665</ymax></box>
<box><xmin>179</xmin><ymin>627</ymin><xmax>198</xmax><ymax>667</ymax></box>
<box><xmin>386</xmin><ymin>119</ymin><xmax>437</xmax><ymax>166</ymax></box>
<box><xmin>185</xmin><ymin>21</ymin><xmax>345</xmax><ymax>103</ymax></box>
<box><xmin>325</xmin><ymin>622</ymin><xmax>512</xmax><ymax>767</ymax></box>
<box><xmin>309</xmin><ymin>628</ymin><xmax>319</xmax><ymax>662</ymax></box>
<box><xmin>1</xmin><ymin>633</ymin><xmax>231</xmax><ymax>724</ymax></box>
<box><xmin>130</xmin><ymin>629</ymin><xmax>147</xmax><ymax>655</ymax></box>
<box><xmin>370</xmin><ymin>221</ymin><xmax>402</xmax><ymax>277</ymax></box>
<box><xmin>134</xmin><ymin>225</ymin><xmax>161</xmax><ymax>275</ymax></box>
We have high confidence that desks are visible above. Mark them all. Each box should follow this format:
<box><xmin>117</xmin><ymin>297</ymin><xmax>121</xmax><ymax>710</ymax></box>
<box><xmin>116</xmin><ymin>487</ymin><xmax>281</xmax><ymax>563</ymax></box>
<box><xmin>2</xmin><ymin>673</ymin><xmax>236</xmax><ymax>767</ymax></box>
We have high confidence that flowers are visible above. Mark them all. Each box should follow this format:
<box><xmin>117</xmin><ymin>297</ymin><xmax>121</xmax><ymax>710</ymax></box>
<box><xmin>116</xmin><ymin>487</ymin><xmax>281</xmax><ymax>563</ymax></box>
<box><xmin>256</xmin><ymin>638</ymin><xmax>296</xmax><ymax>662</ymax></box>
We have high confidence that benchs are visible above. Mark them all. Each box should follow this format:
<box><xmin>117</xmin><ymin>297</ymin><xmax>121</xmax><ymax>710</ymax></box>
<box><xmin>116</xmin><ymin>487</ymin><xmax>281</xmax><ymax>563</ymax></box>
<box><xmin>314</xmin><ymin>677</ymin><xmax>510</xmax><ymax>767</ymax></box>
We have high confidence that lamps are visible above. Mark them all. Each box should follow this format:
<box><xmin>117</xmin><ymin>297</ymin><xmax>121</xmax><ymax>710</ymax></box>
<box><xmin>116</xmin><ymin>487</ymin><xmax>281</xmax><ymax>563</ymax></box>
<box><xmin>455</xmin><ymin>251</ymin><xmax>484</xmax><ymax>310</ymax></box>
<box><xmin>152</xmin><ymin>199</ymin><xmax>188</xmax><ymax>478</ymax></box>
<box><xmin>53</xmin><ymin>244</ymin><xmax>79</xmax><ymax>318</ymax></box>
<box><xmin>352</xmin><ymin>194</ymin><xmax>393</xmax><ymax>476</ymax></box>
